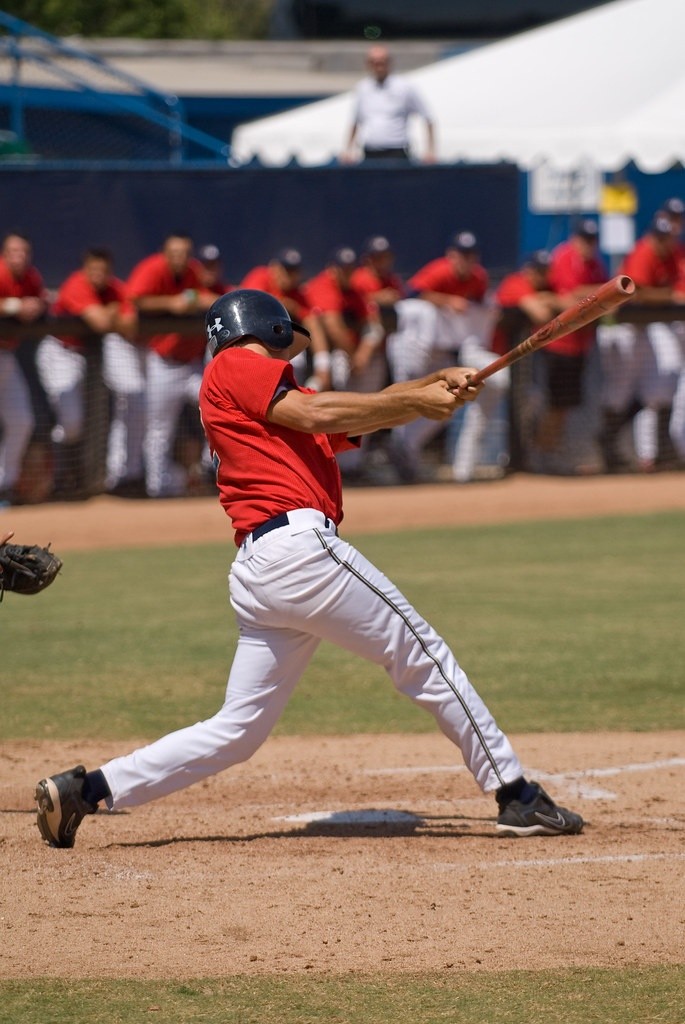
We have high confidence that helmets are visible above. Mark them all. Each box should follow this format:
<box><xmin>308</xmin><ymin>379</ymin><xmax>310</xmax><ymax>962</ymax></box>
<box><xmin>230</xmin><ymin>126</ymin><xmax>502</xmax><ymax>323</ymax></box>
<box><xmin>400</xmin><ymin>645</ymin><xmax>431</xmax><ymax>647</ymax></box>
<box><xmin>204</xmin><ymin>288</ymin><xmax>312</xmax><ymax>363</ymax></box>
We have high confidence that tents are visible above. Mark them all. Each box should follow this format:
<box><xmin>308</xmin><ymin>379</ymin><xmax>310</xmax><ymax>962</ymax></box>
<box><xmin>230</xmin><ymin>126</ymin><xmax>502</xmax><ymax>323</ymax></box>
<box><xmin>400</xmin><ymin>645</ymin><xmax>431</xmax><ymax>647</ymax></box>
<box><xmin>230</xmin><ymin>0</ymin><xmax>685</xmax><ymax>175</ymax></box>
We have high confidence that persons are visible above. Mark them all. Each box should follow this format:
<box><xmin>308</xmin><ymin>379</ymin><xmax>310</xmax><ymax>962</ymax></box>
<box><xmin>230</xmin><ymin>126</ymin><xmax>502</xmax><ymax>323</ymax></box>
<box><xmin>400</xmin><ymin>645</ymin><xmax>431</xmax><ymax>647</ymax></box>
<box><xmin>33</xmin><ymin>286</ymin><xmax>583</xmax><ymax>848</ymax></box>
<box><xmin>0</xmin><ymin>173</ymin><xmax>685</xmax><ymax>505</ymax></box>
<box><xmin>344</xmin><ymin>47</ymin><xmax>436</xmax><ymax>164</ymax></box>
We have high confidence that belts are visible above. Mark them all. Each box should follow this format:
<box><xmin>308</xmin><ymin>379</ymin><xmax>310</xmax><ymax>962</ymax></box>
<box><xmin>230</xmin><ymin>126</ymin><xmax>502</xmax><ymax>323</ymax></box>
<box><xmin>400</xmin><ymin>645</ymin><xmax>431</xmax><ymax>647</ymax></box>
<box><xmin>245</xmin><ymin>511</ymin><xmax>339</xmax><ymax>543</ymax></box>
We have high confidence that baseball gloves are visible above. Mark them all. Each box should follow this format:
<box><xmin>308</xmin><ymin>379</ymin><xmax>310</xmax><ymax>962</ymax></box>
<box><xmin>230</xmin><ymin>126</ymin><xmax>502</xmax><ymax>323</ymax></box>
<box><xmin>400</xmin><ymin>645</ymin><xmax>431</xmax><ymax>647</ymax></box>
<box><xmin>2</xmin><ymin>531</ymin><xmax>63</xmax><ymax>598</ymax></box>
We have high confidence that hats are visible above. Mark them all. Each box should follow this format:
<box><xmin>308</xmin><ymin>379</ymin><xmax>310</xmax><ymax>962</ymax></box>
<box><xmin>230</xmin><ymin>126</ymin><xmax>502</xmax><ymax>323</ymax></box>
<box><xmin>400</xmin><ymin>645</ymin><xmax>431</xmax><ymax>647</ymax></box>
<box><xmin>197</xmin><ymin>244</ymin><xmax>222</xmax><ymax>260</ymax></box>
<box><xmin>368</xmin><ymin>234</ymin><xmax>401</xmax><ymax>252</ymax></box>
<box><xmin>529</xmin><ymin>247</ymin><xmax>552</xmax><ymax>269</ymax></box>
<box><xmin>666</xmin><ymin>196</ymin><xmax>684</xmax><ymax>212</ymax></box>
<box><xmin>273</xmin><ymin>242</ymin><xmax>305</xmax><ymax>266</ymax></box>
<box><xmin>652</xmin><ymin>217</ymin><xmax>672</xmax><ymax>235</ymax></box>
<box><xmin>332</xmin><ymin>246</ymin><xmax>355</xmax><ymax>266</ymax></box>
<box><xmin>447</xmin><ymin>230</ymin><xmax>480</xmax><ymax>250</ymax></box>
<box><xmin>576</xmin><ymin>218</ymin><xmax>600</xmax><ymax>240</ymax></box>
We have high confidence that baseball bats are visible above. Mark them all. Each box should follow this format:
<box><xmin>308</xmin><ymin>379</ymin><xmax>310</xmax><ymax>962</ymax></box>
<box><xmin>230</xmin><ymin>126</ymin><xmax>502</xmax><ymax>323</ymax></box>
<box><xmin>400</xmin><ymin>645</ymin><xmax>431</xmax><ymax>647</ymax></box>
<box><xmin>449</xmin><ymin>275</ymin><xmax>636</xmax><ymax>398</ymax></box>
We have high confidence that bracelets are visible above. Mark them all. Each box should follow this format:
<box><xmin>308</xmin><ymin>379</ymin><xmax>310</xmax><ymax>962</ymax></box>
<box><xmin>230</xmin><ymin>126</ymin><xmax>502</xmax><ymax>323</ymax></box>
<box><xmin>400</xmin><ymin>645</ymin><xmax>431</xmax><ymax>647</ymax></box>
<box><xmin>7</xmin><ymin>298</ymin><xmax>21</xmax><ymax>313</ymax></box>
<box><xmin>184</xmin><ymin>289</ymin><xmax>196</xmax><ymax>307</ymax></box>
<box><xmin>363</xmin><ymin>329</ymin><xmax>382</xmax><ymax>344</ymax></box>
<box><xmin>313</xmin><ymin>351</ymin><xmax>331</xmax><ymax>373</ymax></box>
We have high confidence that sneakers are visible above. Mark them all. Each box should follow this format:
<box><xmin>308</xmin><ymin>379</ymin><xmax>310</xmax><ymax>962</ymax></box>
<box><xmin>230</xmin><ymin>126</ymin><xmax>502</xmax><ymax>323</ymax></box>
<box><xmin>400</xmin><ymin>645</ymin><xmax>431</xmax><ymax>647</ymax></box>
<box><xmin>494</xmin><ymin>781</ymin><xmax>584</xmax><ymax>837</ymax></box>
<box><xmin>32</xmin><ymin>766</ymin><xmax>97</xmax><ymax>848</ymax></box>
